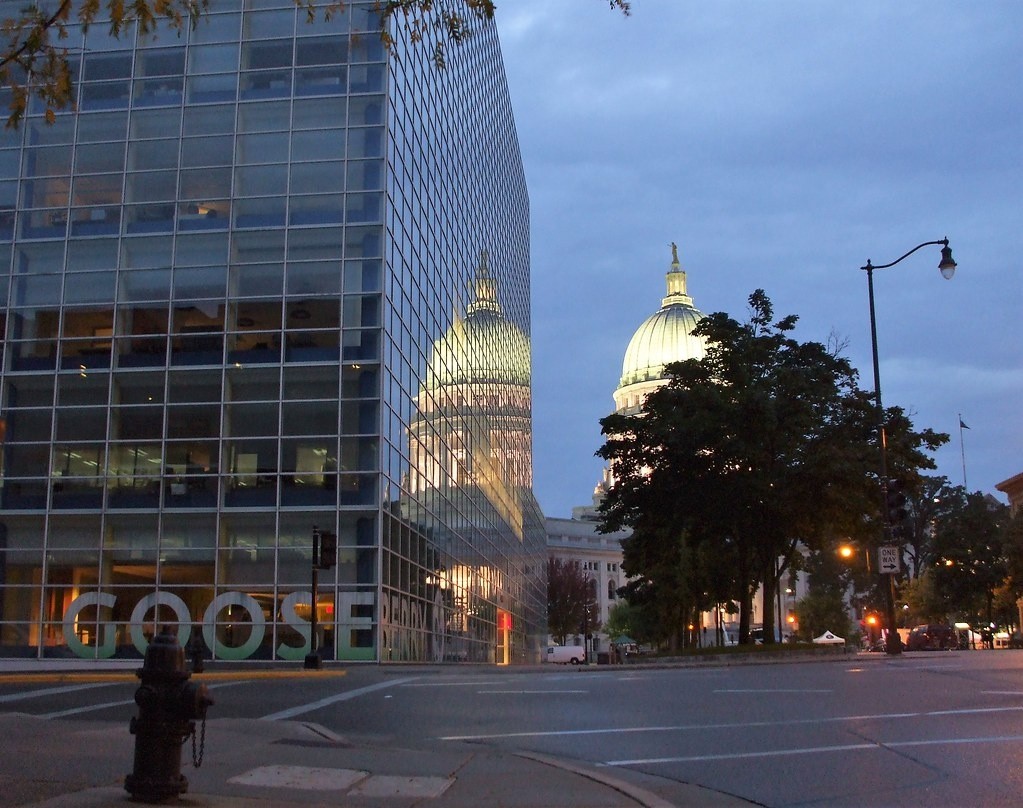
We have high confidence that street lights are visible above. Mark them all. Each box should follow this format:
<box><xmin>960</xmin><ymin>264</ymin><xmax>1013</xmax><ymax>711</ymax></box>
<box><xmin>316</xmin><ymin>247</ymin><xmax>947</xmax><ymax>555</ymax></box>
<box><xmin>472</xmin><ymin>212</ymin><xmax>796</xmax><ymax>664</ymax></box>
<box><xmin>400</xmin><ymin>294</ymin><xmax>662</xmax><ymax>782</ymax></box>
<box><xmin>837</xmin><ymin>538</ymin><xmax>876</xmax><ymax>650</ymax></box>
<box><xmin>858</xmin><ymin>238</ymin><xmax>964</xmax><ymax>655</ymax></box>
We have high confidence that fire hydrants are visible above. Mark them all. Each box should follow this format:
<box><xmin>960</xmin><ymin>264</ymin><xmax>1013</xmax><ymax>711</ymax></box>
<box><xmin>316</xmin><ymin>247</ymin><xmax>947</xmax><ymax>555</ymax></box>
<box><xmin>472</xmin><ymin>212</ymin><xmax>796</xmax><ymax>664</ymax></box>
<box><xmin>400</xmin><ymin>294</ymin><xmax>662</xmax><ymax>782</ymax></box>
<box><xmin>121</xmin><ymin>636</ymin><xmax>221</xmax><ymax>801</ymax></box>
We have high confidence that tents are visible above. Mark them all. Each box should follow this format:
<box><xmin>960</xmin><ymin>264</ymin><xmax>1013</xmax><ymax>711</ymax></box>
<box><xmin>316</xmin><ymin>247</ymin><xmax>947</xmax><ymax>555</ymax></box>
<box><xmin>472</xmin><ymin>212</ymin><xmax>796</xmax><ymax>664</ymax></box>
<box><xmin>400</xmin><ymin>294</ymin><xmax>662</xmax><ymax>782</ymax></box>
<box><xmin>611</xmin><ymin>634</ymin><xmax>637</xmax><ymax>644</ymax></box>
<box><xmin>813</xmin><ymin>630</ymin><xmax>845</xmax><ymax>644</ymax></box>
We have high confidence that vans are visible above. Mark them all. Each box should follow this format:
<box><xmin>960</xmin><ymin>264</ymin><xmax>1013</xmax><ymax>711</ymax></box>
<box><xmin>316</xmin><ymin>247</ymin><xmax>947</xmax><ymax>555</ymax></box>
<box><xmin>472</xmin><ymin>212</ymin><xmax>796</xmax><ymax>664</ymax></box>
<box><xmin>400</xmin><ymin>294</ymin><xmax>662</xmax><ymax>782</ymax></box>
<box><xmin>547</xmin><ymin>645</ymin><xmax>585</xmax><ymax>664</ymax></box>
<box><xmin>904</xmin><ymin>623</ymin><xmax>957</xmax><ymax>650</ymax></box>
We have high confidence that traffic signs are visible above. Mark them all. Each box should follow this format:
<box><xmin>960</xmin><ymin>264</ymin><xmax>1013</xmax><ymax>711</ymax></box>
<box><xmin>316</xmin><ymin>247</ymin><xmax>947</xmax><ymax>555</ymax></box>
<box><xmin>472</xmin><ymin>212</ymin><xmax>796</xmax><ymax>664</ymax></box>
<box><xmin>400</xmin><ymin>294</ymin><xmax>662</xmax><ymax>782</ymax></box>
<box><xmin>877</xmin><ymin>545</ymin><xmax>898</xmax><ymax>575</ymax></box>
